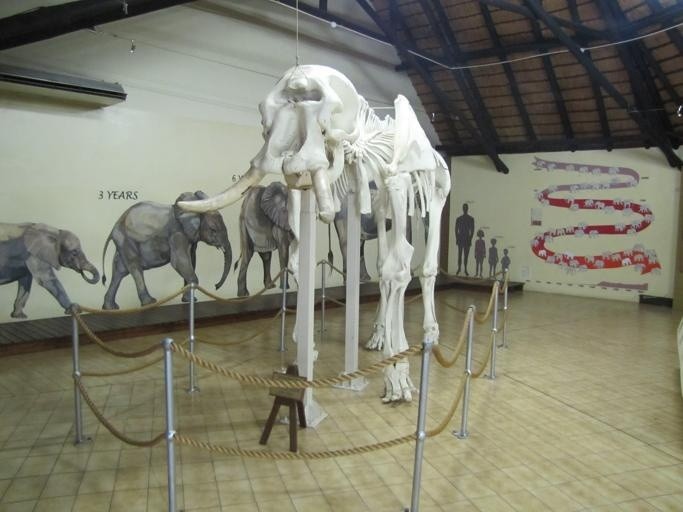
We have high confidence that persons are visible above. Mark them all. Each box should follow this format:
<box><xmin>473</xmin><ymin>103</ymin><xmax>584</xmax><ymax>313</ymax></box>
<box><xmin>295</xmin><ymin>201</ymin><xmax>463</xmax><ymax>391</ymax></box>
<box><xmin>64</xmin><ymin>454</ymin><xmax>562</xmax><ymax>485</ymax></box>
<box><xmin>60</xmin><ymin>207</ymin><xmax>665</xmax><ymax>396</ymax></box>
<box><xmin>454</xmin><ymin>203</ymin><xmax>474</xmax><ymax>276</ymax></box>
<box><xmin>473</xmin><ymin>229</ymin><xmax>486</xmax><ymax>280</ymax></box>
<box><xmin>487</xmin><ymin>238</ymin><xmax>498</xmax><ymax>280</ymax></box>
<box><xmin>500</xmin><ymin>248</ymin><xmax>511</xmax><ymax>278</ymax></box>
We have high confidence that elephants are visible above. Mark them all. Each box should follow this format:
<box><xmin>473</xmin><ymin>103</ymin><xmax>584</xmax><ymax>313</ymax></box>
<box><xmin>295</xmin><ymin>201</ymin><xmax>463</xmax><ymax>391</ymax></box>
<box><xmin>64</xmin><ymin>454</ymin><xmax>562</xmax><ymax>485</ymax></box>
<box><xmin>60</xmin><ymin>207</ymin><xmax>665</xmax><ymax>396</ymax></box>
<box><xmin>101</xmin><ymin>189</ymin><xmax>233</xmax><ymax>311</ymax></box>
<box><xmin>0</xmin><ymin>221</ymin><xmax>100</xmax><ymax>319</ymax></box>
<box><xmin>233</xmin><ymin>181</ymin><xmax>296</xmax><ymax>297</ymax></box>
